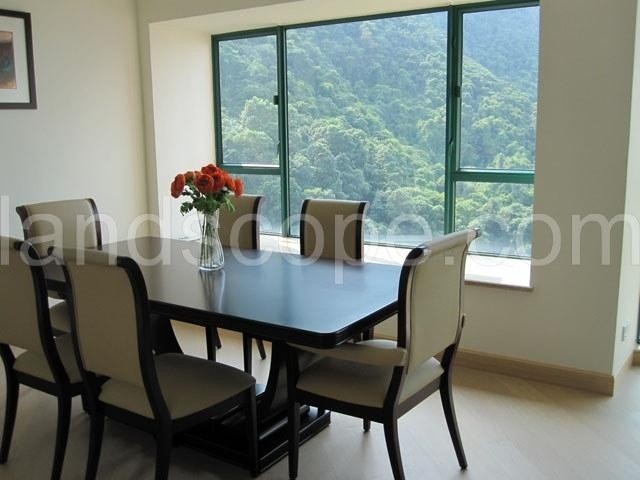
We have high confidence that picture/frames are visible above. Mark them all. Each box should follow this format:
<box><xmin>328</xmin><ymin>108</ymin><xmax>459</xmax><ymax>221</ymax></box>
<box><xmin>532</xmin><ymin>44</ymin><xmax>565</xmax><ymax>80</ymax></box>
<box><xmin>0</xmin><ymin>8</ymin><xmax>37</xmax><ymax>110</ymax></box>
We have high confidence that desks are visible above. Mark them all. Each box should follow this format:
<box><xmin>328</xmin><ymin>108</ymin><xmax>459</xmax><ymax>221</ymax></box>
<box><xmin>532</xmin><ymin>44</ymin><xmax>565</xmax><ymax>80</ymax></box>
<box><xmin>41</xmin><ymin>236</ymin><xmax>400</xmax><ymax>476</ymax></box>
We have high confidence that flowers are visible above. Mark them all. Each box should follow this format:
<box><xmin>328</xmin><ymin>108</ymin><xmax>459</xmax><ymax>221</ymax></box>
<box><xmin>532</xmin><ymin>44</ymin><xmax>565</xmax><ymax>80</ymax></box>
<box><xmin>171</xmin><ymin>165</ymin><xmax>244</xmax><ymax>265</ymax></box>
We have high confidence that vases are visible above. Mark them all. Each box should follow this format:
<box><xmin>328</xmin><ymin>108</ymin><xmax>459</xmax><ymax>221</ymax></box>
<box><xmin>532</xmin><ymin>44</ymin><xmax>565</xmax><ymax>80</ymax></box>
<box><xmin>196</xmin><ymin>208</ymin><xmax>224</xmax><ymax>271</ymax></box>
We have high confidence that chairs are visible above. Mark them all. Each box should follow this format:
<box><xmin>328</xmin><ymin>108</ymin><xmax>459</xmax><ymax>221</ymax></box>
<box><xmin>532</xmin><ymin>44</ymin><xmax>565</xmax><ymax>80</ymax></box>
<box><xmin>0</xmin><ymin>234</ymin><xmax>108</xmax><ymax>480</ymax></box>
<box><xmin>47</xmin><ymin>246</ymin><xmax>260</xmax><ymax>480</ymax></box>
<box><xmin>286</xmin><ymin>224</ymin><xmax>482</xmax><ymax>480</ymax></box>
<box><xmin>286</xmin><ymin>199</ymin><xmax>370</xmax><ymax>412</ymax></box>
<box><xmin>15</xmin><ymin>199</ymin><xmax>102</xmax><ymax>339</ymax></box>
<box><xmin>213</xmin><ymin>194</ymin><xmax>266</xmax><ymax>360</ymax></box>
